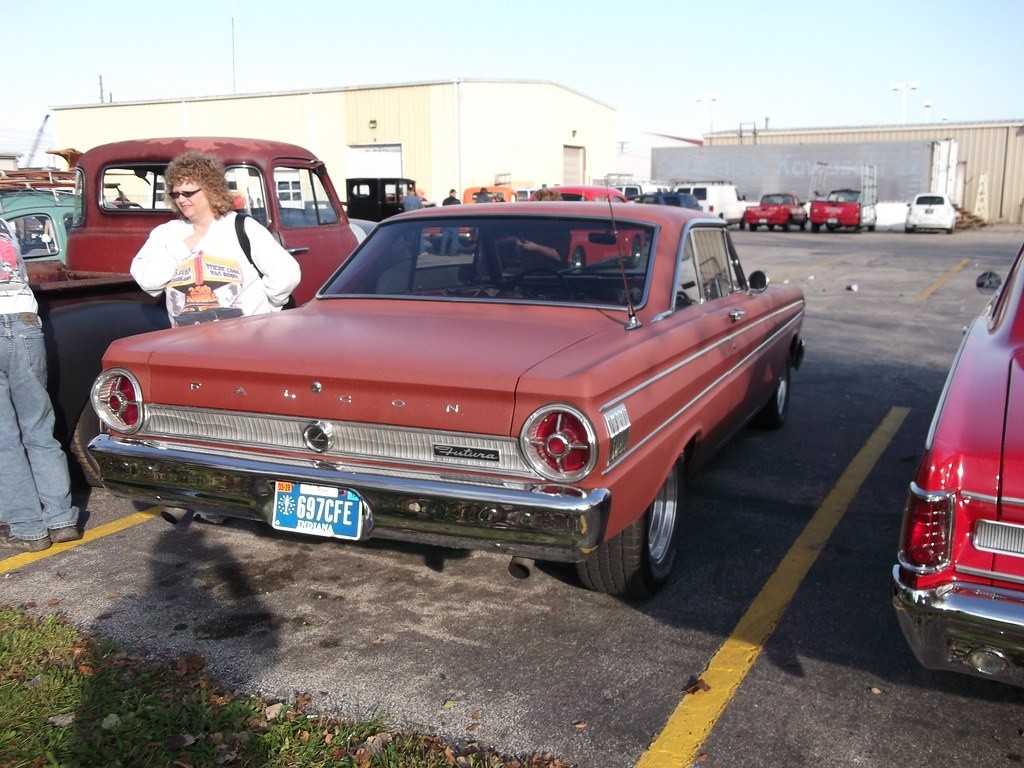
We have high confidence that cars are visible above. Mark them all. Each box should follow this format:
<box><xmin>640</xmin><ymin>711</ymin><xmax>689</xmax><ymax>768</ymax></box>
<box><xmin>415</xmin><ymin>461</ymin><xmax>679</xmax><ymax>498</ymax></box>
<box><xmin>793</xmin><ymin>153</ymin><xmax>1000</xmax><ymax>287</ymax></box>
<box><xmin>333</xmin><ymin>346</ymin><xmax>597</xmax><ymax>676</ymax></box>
<box><xmin>904</xmin><ymin>193</ymin><xmax>955</xmax><ymax>234</ymax></box>
<box><xmin>85</xmin><ymin>201</ymin><xmax>808</xmax><ymax>599</ymax></box>
<box><xmin>0</xmin><ymin>190</ymin><xmax>124</xmax><ymax>263</ymax></box>
<box><xmin>891</xmin><ymin>243</ymin><xmax>1024</xmax><ymax>689</ymax></box>
<box><xmin>528</xmin><ymin>185</ymin><xmax>647</xmax><ymax>274</ymax></box>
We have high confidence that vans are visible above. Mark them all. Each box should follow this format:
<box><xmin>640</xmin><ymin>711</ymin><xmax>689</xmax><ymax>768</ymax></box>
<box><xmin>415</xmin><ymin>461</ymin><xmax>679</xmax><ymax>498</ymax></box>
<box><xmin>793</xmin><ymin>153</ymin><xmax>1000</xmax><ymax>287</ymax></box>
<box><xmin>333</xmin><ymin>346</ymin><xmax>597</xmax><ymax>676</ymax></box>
<box><xmin>674</xmin><ymin>182</ymin><xmax>751</xmax><ymax>229</ymax></box>
<box><xmin>616</xmin><ymin>184</ymin><xmax>673</xmax><ymax>196</ymax></box>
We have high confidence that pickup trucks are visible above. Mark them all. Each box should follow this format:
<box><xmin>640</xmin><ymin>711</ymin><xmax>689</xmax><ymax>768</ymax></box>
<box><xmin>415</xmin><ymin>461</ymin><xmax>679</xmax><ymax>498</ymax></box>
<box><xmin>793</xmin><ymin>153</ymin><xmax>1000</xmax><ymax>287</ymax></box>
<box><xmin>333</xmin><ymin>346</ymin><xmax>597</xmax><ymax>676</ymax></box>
<box><xmin>744</xmin><ymin>192</ymin><xmax>807</xmax><ymax>231</ymax></box>
<box><xmin>304</xmin><ymin>178</ymin><xmax>424</xmax><ymax>221</ymax></box>
<box><xmin>424</xmin><ymin>186</ymin><xmax>521</xmax><ymax>246</ymax></box>
<box><xmin>808</xmin><ymin>189</ymin><xmax>877</xmax><ymax>233</ymax></box>
<box><xmin>29</xmin><ymin>136</ymin><xmax>432</xmax><ymax>451</ymax></box>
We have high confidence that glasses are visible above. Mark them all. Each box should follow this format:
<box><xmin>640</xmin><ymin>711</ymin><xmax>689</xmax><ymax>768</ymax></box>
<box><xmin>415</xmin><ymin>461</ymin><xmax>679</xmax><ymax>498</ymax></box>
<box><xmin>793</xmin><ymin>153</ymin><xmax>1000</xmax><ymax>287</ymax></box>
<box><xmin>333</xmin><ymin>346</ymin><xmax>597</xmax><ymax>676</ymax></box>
<box><xmin>169</xmin><ymin>187</ymin><xmax>202</xmax><ymax>199</ymax></box>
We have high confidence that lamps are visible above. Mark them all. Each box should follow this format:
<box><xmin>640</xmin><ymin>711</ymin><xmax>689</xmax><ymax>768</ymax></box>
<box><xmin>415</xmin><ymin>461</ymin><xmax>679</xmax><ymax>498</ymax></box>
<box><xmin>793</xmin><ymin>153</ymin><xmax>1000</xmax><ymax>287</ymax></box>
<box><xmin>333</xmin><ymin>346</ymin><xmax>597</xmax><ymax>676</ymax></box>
<box><xmin>369</xmin><ymin>120</ymin><xmax>377</xmax><ymax>128</ymax></box>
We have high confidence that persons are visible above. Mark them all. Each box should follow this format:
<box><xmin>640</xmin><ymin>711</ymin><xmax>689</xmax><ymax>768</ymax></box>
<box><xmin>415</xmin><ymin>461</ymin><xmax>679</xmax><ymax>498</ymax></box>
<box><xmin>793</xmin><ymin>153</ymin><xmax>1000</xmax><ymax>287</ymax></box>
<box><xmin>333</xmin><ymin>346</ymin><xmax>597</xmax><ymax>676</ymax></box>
<box><xmin>0</xmin><ymin>213</ymin><xmax>82</xmax><ymax>552</ymax></box>
<box><xmin>475</xmin><ymin>187</ymin><xmax>507</xmax><ymax>202</ymax></box>
<box><xmin>131</xmin><ymin>150</ymin><xmax>302</xmax><ymax>324</ymax></box>
<box><xmin>401</xmin><ymin>184</ymin><xmax>430</xmax><ymax>257</ymax></box>
<box><xmin>440</xmin><ymin>188</ymin><xmax>460</xmax><ymax>257</ymax></box>
<box><xmin>516</xmin><ymin>190</ymin><xmax>571</xmax><ymax>273</ymax></box>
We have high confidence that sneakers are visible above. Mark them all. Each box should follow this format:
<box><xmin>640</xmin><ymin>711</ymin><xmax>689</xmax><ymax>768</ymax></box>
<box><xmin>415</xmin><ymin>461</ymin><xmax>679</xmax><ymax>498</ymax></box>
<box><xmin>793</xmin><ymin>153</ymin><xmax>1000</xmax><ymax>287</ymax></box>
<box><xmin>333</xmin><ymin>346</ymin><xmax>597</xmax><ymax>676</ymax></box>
<box><xmin>48</xmin><ymin>526</ymin><xmax>78</xmax><ymax>542</ymax></box>
<box><xmin>0</xmin><ymin>524</ymin><xmax>50</xmax><ymax>552</ymax></box>
